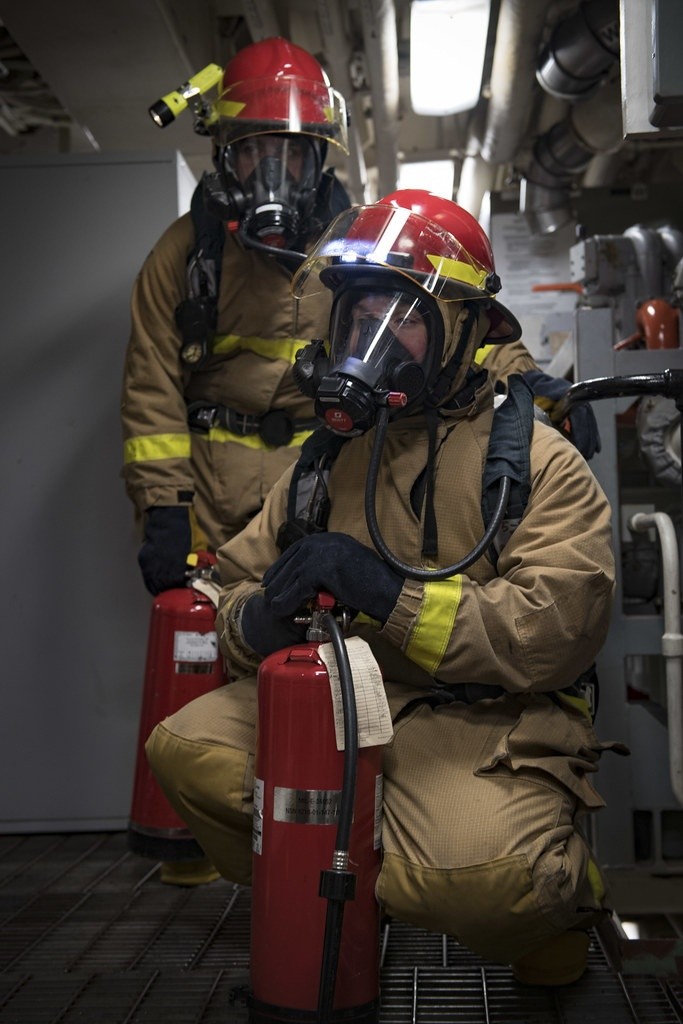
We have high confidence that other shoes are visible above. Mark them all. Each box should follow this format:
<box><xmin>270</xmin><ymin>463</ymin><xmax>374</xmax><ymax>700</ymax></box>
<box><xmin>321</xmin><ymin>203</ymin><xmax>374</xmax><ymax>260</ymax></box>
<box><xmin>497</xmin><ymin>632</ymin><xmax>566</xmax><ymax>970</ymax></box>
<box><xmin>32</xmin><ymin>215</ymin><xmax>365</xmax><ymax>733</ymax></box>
<box><xmin>512</xmin><ymin>857</ymin><xmax>607</xmax><ymax>990</ymax></box>
<box><xmin>156</xmin><ymin>841</ymin><xmax>221</xmax><ymax>884</ymax></box>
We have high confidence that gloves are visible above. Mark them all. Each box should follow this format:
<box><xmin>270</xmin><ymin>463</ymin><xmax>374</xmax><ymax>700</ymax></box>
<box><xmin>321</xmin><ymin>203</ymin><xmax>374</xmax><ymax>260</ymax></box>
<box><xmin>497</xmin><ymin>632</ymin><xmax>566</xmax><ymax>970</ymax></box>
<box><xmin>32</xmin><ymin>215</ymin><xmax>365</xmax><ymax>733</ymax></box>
<box><xmin>137</xmin><ymin>505</ymin><xmax>193</xmax><ymax>597</ymax></box>
<box><xmin>520</xmin><ymin>367</ymin><xmax>601</xmax><ymax>461</ymax></box>
<box><xmin>243</xmin><ymin>517</ymin><xmax>406</xmax><ymax>655</ymax></box>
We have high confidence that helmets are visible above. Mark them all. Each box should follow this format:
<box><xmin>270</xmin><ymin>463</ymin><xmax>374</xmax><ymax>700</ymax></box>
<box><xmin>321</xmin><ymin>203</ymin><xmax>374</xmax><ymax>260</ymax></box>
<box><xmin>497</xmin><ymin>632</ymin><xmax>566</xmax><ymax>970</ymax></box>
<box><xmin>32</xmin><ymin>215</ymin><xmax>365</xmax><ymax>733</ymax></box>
<box><xmin>215</xmin><ymin>36</ymin><xmax>341</xmax><ymax>133</ymax></box>
<box><xmin>319</xmin><ymin>188</ymin><xmax>502</xmax><ymax>297</ymax></box>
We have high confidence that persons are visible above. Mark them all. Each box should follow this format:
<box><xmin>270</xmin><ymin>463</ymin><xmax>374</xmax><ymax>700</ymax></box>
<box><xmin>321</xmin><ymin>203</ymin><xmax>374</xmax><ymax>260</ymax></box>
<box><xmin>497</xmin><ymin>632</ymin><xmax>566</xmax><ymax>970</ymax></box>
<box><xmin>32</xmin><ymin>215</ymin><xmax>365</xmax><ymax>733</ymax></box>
<box><xmin>121</xmin><ymin>39</ymin><xmax>617</xmax><ymax>1001</ymax></box>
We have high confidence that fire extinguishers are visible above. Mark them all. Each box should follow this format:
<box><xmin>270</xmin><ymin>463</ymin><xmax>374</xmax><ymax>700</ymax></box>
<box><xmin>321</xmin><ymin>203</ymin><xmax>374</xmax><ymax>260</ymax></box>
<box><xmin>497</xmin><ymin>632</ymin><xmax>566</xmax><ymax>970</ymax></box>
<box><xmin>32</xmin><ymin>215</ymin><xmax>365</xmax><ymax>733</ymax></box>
<box><xmin>248</xmin><ymin>593</ymin><xmax>389</xmax><ymax>1024</ymax></box>
<box><xmin>124</xmin><ymin>548</ymin><xmax>229</xmax><ymax>862</ymax></box>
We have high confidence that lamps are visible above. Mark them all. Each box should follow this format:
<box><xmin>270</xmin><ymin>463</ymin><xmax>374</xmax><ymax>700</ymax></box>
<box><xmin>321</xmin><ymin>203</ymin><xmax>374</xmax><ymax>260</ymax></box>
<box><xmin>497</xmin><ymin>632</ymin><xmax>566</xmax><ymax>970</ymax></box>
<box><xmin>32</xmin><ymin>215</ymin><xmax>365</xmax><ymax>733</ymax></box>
<box><xmin>408</xmin><ymin>0</ymin><xmax>491</xmax><ymax>116</ymax></box>
